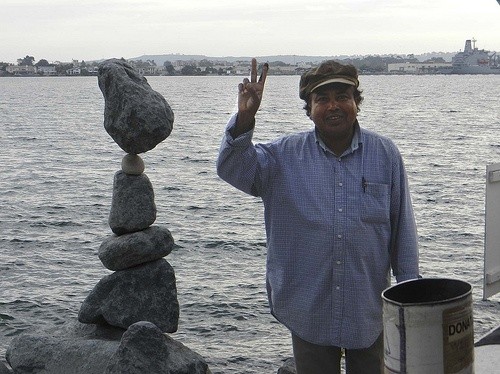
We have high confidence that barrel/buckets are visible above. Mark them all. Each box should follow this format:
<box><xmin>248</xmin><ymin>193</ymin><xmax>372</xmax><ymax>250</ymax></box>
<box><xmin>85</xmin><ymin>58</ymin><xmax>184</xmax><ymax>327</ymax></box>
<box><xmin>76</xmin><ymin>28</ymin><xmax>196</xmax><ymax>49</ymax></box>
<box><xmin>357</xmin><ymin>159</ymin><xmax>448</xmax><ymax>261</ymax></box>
<box><xmin>378</xmin><ymin>278</ymin><xmax>477</xmax><ymax>373</ymax></box>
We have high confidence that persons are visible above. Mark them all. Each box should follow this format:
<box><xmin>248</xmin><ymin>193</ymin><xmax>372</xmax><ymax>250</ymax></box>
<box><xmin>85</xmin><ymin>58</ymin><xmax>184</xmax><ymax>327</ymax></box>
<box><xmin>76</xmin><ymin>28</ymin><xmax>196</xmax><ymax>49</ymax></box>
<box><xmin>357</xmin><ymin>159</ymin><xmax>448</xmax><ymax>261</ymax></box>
<box><xmin>214</xmin><ymin>56</ymin><xmax>422</xmax><ymax>374</ymax></box>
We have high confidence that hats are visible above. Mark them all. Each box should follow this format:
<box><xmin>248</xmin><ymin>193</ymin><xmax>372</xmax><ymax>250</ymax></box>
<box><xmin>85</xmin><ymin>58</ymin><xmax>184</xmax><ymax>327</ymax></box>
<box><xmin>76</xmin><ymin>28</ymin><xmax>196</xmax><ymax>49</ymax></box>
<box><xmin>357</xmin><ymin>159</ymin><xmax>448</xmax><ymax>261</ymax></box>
<box><xmin>299</xmin><ymin>58</ymin><xmax>359</xmax><ymax>100</ymax></box>
